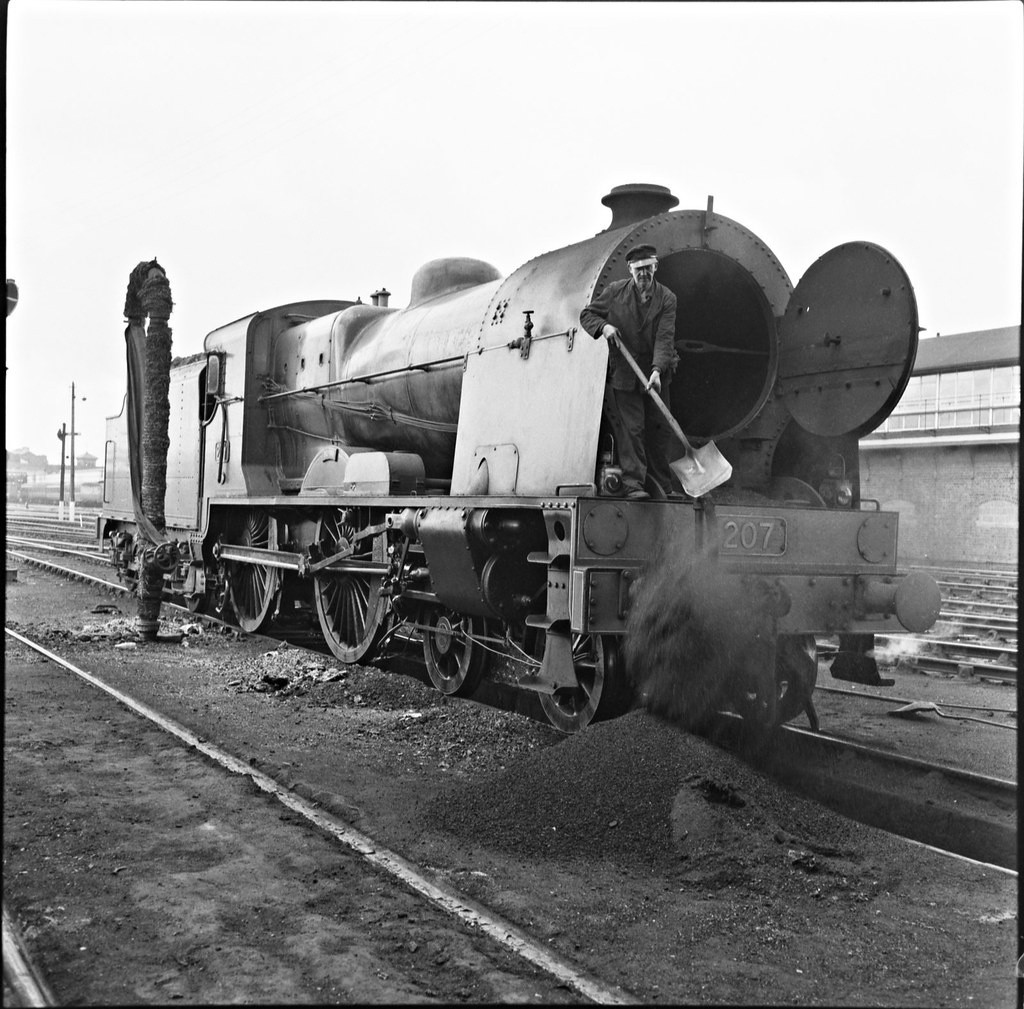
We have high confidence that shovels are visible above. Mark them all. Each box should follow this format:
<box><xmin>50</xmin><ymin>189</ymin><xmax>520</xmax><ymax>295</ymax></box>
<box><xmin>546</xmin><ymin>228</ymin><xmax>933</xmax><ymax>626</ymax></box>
<box><xmin>886</xmin><ymin>700</ymin><xmax>1018</xmax><ymax>730</ymax></box>
<box><xmin>614</xmin><ymin>335</ymin><xmax>734</xmax><ymax>500</ymax></box>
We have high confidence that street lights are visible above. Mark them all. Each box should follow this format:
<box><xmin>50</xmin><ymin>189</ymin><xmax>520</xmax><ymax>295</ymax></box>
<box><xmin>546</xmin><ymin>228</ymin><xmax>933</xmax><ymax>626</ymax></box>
<box><xmin>69</xmin><ymin>396</ymin><xmax>87</xmax><ymax>523</ymax></box>
<box><xmin>60</xmin><ymin>432</ymin><xmax>81</xmax><ymax>520</ymax></box>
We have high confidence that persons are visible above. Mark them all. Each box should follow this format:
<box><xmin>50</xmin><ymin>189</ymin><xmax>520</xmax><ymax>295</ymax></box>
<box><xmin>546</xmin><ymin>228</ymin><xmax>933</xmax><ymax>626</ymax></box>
<box><xmin>577</xmin><ymin>244</ymin><xmax>682</xmax><ymax>499</ymax></box>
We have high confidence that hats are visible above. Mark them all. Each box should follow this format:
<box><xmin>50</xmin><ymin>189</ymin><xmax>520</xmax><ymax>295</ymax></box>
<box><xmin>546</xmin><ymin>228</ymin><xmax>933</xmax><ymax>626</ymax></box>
<box><xmin>625</xmin><ymin>243</ymin><xmax>658</xmax><ymax>269</ymax></box>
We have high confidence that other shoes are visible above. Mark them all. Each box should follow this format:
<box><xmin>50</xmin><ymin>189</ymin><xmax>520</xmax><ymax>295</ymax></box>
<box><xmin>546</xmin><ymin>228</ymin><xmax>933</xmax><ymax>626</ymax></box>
<box><xmin>626</xmin><ymin>488</ymin><xmax>651</xmax><ymax>500</ymax></box>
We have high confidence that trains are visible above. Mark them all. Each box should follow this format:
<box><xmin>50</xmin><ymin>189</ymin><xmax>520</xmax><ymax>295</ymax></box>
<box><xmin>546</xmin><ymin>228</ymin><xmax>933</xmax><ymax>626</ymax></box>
<box><xmin>93</xmin><ymin>184</ymin><xmax>942</xmax><ymax>746</ymax></box>
